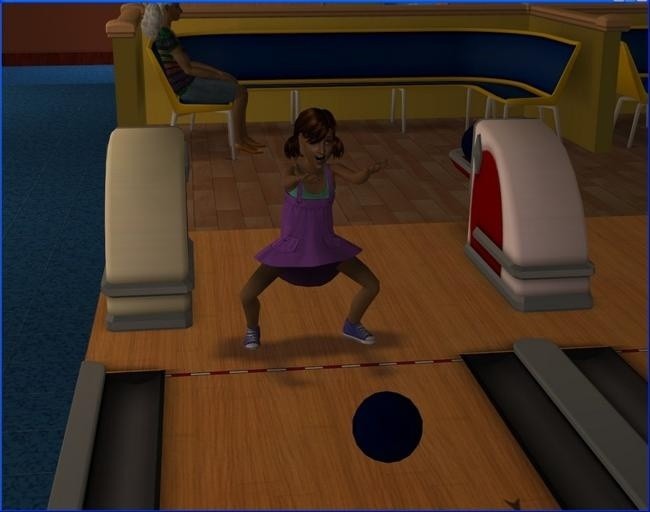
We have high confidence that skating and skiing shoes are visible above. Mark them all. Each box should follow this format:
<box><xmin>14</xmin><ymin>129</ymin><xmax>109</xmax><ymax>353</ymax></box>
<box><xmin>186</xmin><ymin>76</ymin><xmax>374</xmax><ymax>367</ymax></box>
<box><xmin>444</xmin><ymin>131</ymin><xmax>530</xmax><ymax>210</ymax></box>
<box><xmin>243</xmin><ymin>326</ymin><xmax>261</xmax><ymax>350</ymax></box>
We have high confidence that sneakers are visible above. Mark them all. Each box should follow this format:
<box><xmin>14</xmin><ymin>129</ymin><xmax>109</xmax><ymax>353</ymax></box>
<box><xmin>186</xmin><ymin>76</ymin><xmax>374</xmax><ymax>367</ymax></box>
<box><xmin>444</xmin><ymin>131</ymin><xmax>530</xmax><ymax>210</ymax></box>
<box><xmin>342</xmin><ymin>321</ymin><xmax>377</xmax><ymax>345</ymax></box>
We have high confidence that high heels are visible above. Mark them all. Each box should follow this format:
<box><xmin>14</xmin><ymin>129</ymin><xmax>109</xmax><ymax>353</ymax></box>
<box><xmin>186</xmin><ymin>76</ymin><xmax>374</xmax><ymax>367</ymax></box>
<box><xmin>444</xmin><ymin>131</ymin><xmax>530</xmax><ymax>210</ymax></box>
<box><xmin>233</xmin><ymin>134</ymin><xmax>266</xmax><ymax>155</ymax></box>
